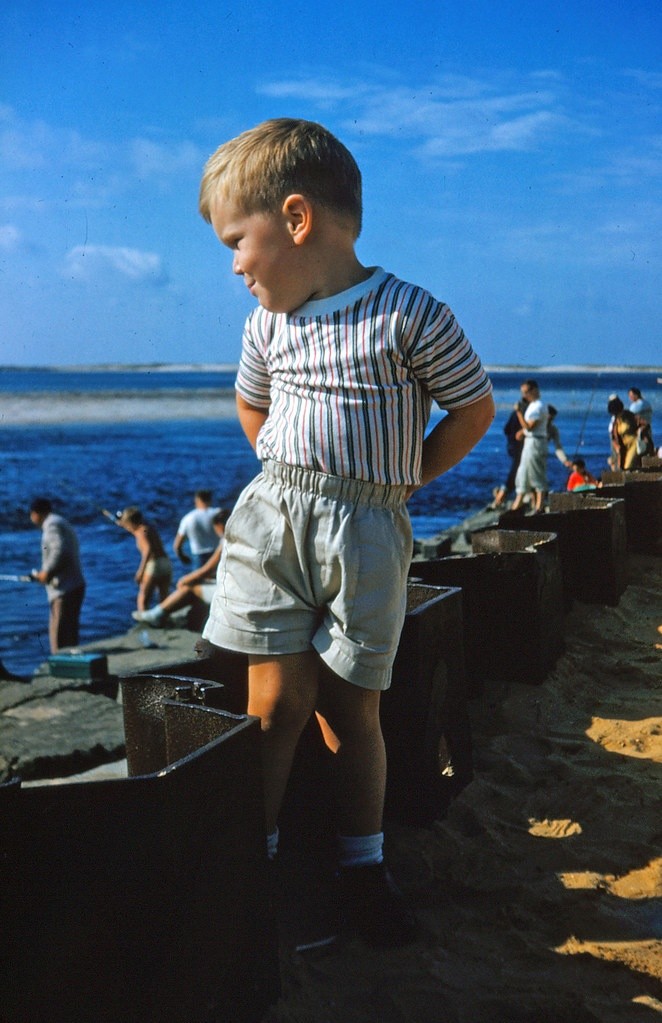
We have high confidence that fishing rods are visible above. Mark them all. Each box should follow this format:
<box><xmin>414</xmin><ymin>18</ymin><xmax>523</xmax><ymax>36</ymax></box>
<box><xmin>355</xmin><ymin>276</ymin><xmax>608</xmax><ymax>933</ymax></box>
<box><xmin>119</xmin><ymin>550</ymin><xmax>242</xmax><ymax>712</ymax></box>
<box><xmin>0</xmin><ymin>568</ymin><xmax>51</xmax><ymax>584</ymax></box>
<box><xmin>573</xmin><ymin>371</ymin><xmax>600</xmax><ymax>461</ymax></box>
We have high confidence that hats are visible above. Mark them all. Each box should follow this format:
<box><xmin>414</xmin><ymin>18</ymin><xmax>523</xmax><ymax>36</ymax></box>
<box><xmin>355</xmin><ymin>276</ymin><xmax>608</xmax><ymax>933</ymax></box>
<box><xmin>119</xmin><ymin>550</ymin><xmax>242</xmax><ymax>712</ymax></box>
<box><xmin>608</xmin><ymin>397</ymin><xmax>624</xmax><ymax>413</ymax></box>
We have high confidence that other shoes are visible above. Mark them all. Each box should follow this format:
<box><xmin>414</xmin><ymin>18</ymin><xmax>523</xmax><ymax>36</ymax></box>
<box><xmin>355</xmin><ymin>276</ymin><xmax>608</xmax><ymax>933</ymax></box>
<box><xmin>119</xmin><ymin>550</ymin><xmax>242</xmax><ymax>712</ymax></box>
<box><xmin>131</xmin><ymin>609</ymin><xmax>163</xmax><ymax>628</ymax></box>
<box><xmin>336</xmin><ymin>859</ymin><xmax>411</xmax><ymax>941</ymax></box>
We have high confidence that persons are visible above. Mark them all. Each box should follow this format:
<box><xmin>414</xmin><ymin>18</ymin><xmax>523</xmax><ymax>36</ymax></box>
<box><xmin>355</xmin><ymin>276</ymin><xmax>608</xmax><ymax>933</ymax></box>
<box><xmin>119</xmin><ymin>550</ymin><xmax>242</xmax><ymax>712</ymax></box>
<box><xmin>132</xmin><ymin>510</ymin><xmax>232</xmax><ymax>627</ymax></box>
<box><xmin>567</xmin><ymin>460</ymin><xmax>597</xmax><ymax>493</ymax></box>
<box><xmin>173</xmin><ymin>492</ymin><xmax>223</xmax><ymax>572</ymax></box>
<box><xmin>30</xmin><ymin>499</ymin><xmax>86</xmax><ymax>653</ymax></box>
<box><xmin>485</xmin><ymin>380</ymin><xmax>573</xmax><ymax>517</ymax></box>
<box><xmin>123</xmin><ymin>507</ymin><xmax>173</xmax><ymax>611</ymax></box>
<box><xmin>199</xmin><ymin>120</ymin><xmax>496</xmax><ymax>941</ymax></box>
<box><xmin>608</xmin><ymin>387</ymin><xmax>655</xmax><ymax>472</ymax></box>
